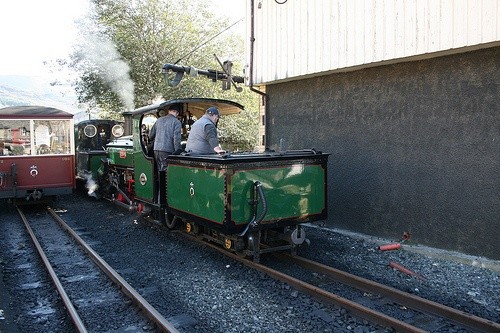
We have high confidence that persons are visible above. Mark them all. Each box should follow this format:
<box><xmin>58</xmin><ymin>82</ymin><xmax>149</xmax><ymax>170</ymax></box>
<box><xmin>147</xmin><ymin>105</ymin><xmax>184</xmax><ymax>202</ymax></box>
<box><xmin>184</xmin><ymin>106</ymin><xmax>229</xmax><ymax>155</ymax></box>
<box><xmin>35</xmin><ymin>121</ymin><xmax>55</xmax><ymax>149</ymax></box>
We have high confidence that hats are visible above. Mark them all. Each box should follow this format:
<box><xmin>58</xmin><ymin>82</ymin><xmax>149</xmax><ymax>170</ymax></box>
<box><xmin>168</xmin><ymin>104</ymin><xmax>179</xmax><ymax>113</ymax></box>
<box><xmin>206</xmin><ymin>106</ymin><xmax>219</xmax><ymax>116</ymax></box>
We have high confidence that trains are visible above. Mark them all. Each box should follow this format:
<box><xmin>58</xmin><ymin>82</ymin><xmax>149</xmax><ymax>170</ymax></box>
<box><xmin>0</xmin><ymin>105</ymin><xmax>75</xmax><ymax>210</ymax></box>
<box><xmin>77</xmin><ymin>96</ymin><xmax>333</xmax><ymax>261</ymax></box>
